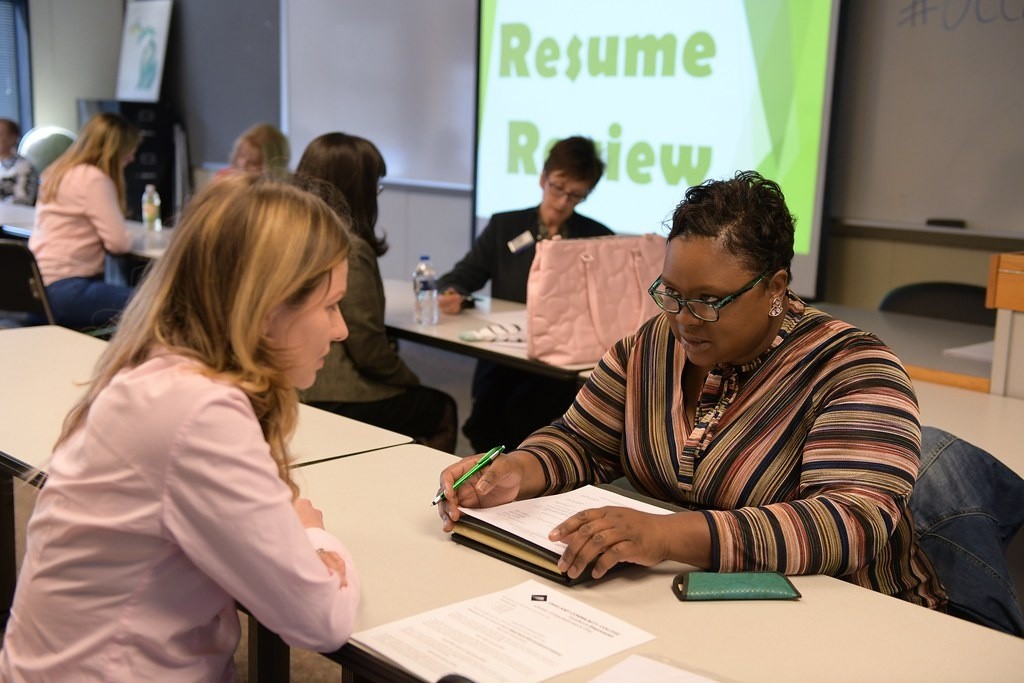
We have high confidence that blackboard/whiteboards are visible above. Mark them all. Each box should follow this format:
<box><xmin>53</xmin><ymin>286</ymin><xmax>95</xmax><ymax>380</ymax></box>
<box><xmin>281</xmin><ymin>0</ymin><xmax>1024</xmax><ymax>252</ymax></box>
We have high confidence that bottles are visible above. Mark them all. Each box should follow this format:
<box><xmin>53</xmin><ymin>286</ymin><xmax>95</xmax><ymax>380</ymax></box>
<box><xmin>412</xmin><ymin>255</ymin><xmax>437</xmax><ymax>324</ymax></box>
<box><xmin>141</xmin><ymin>185</ymin><xmax>161</xmax><ymax>232</ymax></box>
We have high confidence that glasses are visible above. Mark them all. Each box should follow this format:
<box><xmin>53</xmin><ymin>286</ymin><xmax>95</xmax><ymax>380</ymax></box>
<box><xmin>648</xmin><ymin>251</ymin><xmax>798</xmax><ymax>322</ymax></box>
<box><xmin>545</xmin><ymin>174</ymin><xmax>588</xmax><ymax>204</ymax></box>
<box><xmin>375</xmin><ymin>184</ymin><xmax>384</xmax><ymax>196</ymax></box>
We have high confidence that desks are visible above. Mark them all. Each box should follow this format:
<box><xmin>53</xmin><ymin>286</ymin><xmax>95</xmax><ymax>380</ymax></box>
<box><xmin>812</xmin><ymin>302</ymin><xmax>997</xmax><ymax>378</ymax></box>
<box><xmin>0</xmin><ymin>325</ymin><xmax>413</xmax><ymax>638</ymax></box>
<box><xmin>244</xmin><ymin>324</ymin><xmax>1024</xmax><ymax>683</ymax></box>
<box><xmin>0</xmin><ymin>199</ymin><xmax>174</xmax><ymax>282</ymax></box>
<box><xmin>372</xmin><ymin>282</ymin><xmax>595</xmax><ymax>386</ymax></box>
<box><xmin>910</xmin><ymin>379</ymin><xmax>1024</xmax><ymax>481</ymax></box>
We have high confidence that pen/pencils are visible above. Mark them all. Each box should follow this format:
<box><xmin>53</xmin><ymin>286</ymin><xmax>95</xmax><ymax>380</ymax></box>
<box><xmin>450</xmin><ymin>291</ymin><xmax>482</xmax><ymax>302</ymax></box>
<box><xmin>430</xmin><ymin>445</ymin><xmax>506</xmax><ymax>506</ymax></box>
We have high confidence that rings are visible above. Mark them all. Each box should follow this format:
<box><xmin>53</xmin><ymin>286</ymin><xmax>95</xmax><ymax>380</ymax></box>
<box><xmin>317</xmin><ymin>548</ymin><xmax>324</xmax><ymax>553</ymax></box>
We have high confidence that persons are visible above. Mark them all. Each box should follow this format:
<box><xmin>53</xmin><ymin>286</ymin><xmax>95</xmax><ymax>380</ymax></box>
<box><xmin>229</xmin><ymin>123</ymin><xmax>290</xmax><ymax>171</ymax></box>
<box><xmin>436</xmin><ymin>136</ymin><xmax>616</xmax><ymax>453</ymax></box>
<box><xmin>0</xmin><ymin>171</ymin><xmax>362</xmax><ymax>683</ymax></box>
<box><xmin>436</xmin><ymin>169</ymin><xmax>950</xmax><ymax>613</ymax></box>
<box><xmin>294</xmin><ymin>133</ymin><xmax>461</xmax><ymax>453</ymax></box>
<box><xmin>0</xmin><ymin>119</ymin><xmax>38</xmax><ymax>205</ymax></box>
<box><xmin>27</xmin><ymin>110</ymin><xmax>137</xmax><ymax>327</ymax></box>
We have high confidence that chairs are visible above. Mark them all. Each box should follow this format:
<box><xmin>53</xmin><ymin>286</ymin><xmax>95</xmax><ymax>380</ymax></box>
<box><xmin>0</xmin><ymin>240</ymin><xmax>120</xmax><ymax>340</ymax></box>
<box><xmin>878</xmin><ymin>281</ymin><xmax>995</xmax><ymax>326</ymax></box>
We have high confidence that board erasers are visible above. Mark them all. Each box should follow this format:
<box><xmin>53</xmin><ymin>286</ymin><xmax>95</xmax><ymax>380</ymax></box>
<box><xmin>927</xmin><ymin>218</ymin><xmax>967</xmax><ymax>229</ymax></box>
